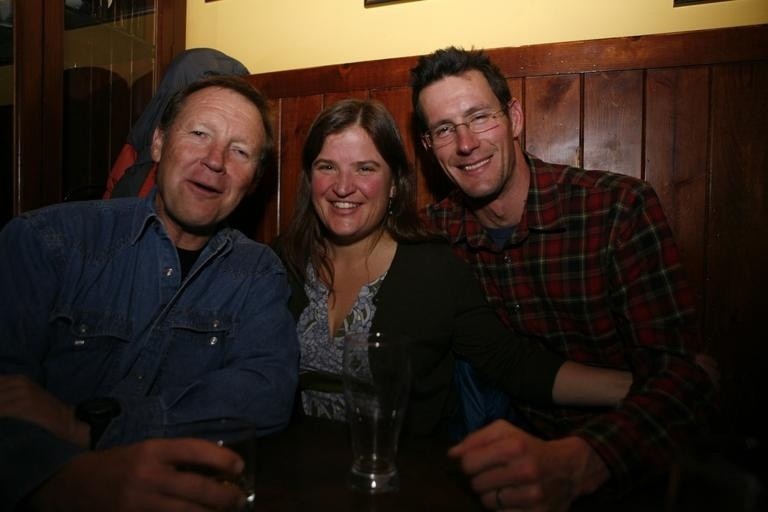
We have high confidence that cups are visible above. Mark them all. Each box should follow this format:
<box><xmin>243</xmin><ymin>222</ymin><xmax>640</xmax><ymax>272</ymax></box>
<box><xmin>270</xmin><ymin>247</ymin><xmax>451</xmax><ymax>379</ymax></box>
<box><xmin>181</xmin><ymin>434</ymin><xmax>257</xmax><ymax>505</ymax></box>
<box><xmin>343</xmin><ymin>332</ymin><xmax>411</xmax><ymax>495</ymax></box>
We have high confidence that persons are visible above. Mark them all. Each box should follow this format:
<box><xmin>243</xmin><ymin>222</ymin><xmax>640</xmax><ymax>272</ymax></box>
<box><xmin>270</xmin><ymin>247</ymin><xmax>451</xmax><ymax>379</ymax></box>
<box><xmin>266</xmin><ymin>97</ymin><xmax>724</xmax><ymax>448</ymax></box>
<box><xmin>1</xmin><ymin>71</ymin><xmax>298</xmax><ymax>510</ymax></box>
<box><xmin>404</xmin><ymin>46</ymin><xmax>767</xmax><ymax>511</ymax></box>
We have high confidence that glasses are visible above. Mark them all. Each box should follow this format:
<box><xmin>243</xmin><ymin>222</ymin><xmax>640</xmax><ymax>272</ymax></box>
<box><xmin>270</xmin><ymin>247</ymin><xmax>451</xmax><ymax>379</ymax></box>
<box><xmin>416</xmin><ymin>98</ymin><xmax>513</xmax><ymax>151</ymax></box>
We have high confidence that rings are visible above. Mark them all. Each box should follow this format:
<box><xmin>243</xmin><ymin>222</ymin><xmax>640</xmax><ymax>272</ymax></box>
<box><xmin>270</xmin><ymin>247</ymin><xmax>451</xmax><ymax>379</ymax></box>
<box><xmin>495</xmin><ymin>489</ymin><xmax>504</xmax><ymax>511</ymax></box>
